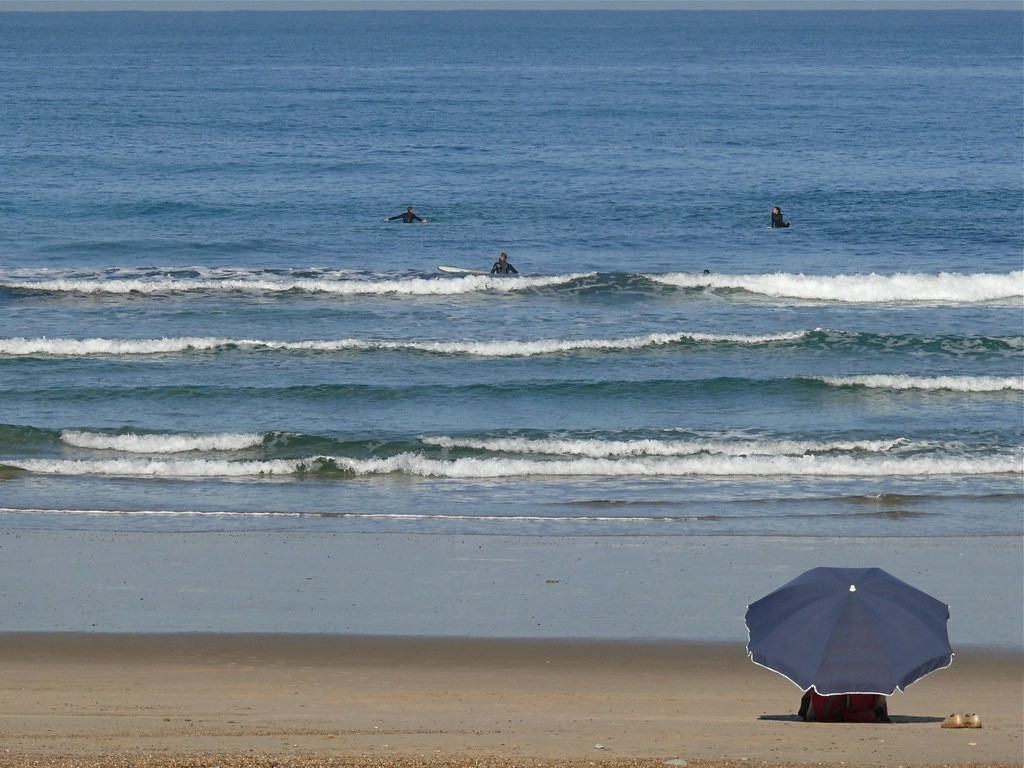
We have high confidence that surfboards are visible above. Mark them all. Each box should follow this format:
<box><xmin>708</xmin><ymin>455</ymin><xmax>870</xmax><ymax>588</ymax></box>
<box><xmin>437</xmin><ymin>266</ymin><xmax>489</xmax><ymax>274</ymax></box>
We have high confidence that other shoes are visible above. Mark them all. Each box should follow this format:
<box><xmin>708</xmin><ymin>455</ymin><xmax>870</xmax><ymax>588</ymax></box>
<box><xmin>940</xmin><ymin>714</ymin><xmax>964</xmax><ymax>728</ymax></box>
<box><xmin>962</xmin><ymin>713</ymin><xmax>982</xmax><ymax>728</ymax></box>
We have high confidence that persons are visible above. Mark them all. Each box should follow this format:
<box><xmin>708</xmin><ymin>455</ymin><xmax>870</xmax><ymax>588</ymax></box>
<box><xmin>384</xmin><ymin>207</ymin><xmax>428</xmax><ymax>223</ymax></box>
<box><xmin>490</xmin><ymin>253</ymin><xmax>518</xmax><ymax>274</ymax></box>
<box><xmin>771</xmin><ymin>207</ymin><xmax>790</xmax><ymax>227</ymax></box>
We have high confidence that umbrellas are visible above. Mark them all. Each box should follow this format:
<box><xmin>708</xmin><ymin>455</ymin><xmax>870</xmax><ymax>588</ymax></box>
<box><xmin>743</xmin><ymin>566</ymin><xmax>955</xmax><ymax>696</ymax></box>
